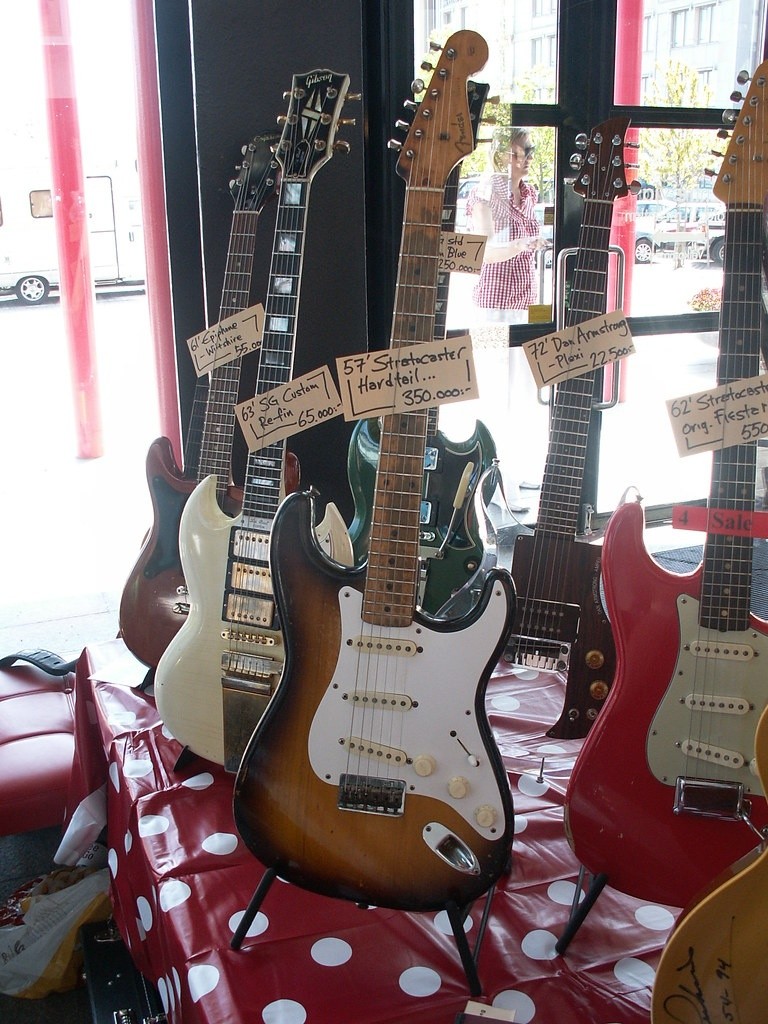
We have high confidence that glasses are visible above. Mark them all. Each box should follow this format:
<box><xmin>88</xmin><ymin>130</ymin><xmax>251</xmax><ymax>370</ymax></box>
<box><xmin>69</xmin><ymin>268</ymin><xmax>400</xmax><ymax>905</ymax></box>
<box><xmin>505</xmin><ymin>145</ymin><xmax>536</xmax><ymax>159</ymax></box>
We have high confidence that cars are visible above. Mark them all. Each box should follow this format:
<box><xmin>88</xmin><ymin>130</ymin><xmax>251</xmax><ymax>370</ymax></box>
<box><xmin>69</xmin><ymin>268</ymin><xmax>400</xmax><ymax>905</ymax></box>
<box><xmin>454</xmin><ymin>175</ymin><xmax>724</xmax><ymax>266</ymax></box>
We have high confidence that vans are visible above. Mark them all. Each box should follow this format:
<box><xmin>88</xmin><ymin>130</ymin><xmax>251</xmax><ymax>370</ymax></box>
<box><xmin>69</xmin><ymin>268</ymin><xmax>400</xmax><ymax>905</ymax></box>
<box><xmin>1</xmin><ymin>171</ymin><xmax>145</xmax><ymax>305</ymax></box>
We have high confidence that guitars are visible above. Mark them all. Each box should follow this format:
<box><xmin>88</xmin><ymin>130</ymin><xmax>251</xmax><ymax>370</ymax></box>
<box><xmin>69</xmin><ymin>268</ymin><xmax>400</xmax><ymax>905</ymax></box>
<box><xmin>120</xmin><ymin>135</ymin><xmax>283</xmax><ymax>663</ymax></box>
<box><xmin>566</xmin><ymin>62</ymin><xmax>767</xmax><ymax>904</ymax></box>
<box><xmin>346</xmin><ymin>81</ymin><xmax>497</xmax><ymax>617</ymax></box>
<box><xmin>154</xmin><ymin>71</ymin><xmax>357</xmax><ymax>768</ymax></box>
<box><xmin>232</xmin><ymin>28</ymin><xmax>518</xmax><ymax>907</ymax></box>
<box><xmin>651</xmin><ymin>707</ymin><xmax>767</xmax><ymax>1023</ymax></box>
<box><xmin>488</xmin><ymin>110</ymin><xmax>641</xmax><ymax>735</ymax></box>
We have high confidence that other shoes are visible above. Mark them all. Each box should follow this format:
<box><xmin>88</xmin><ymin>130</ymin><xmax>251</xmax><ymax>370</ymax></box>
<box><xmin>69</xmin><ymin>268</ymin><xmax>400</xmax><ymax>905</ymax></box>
<box><xmin>516</xmin><ymin>479</ymin><xmax>542</xmax><ymax>491</ymax></box>
<box><xmin>489</xmin><ymin>497</ymin><xmax>530</xmax><ymax>512</ymax></box>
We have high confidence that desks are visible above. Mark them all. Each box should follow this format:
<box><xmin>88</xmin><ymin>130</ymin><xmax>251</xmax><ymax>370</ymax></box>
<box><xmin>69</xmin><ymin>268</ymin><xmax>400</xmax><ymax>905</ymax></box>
<box><xmin>54</xmin><ymin>639</ymin><xmax>682</xmax><ymax>1024</ymax></box>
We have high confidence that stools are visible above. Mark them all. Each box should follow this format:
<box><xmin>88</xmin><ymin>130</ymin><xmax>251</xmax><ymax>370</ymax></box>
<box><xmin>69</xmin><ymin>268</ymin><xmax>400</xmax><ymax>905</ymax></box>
<box><xmin>0</xmin><ymin>665</ymin><xmax>76</xmax><ymax>837</ymax></box>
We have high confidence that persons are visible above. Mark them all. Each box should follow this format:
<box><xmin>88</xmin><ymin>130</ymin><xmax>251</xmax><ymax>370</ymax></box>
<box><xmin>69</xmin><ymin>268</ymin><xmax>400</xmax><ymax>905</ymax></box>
<box><xmin>466</xmin><ymin>129</ymin><xmax>550</xmax><ymax>515</ymax></box>
